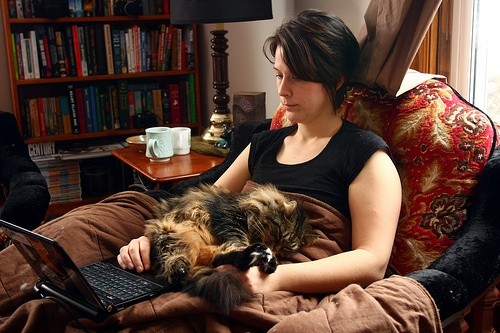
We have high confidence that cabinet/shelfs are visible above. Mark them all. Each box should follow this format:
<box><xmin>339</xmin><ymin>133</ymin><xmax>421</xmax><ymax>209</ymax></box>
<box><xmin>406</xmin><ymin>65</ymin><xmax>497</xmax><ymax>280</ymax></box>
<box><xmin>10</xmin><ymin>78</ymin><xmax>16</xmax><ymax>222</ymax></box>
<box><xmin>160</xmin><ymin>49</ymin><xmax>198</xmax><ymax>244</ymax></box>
<box><xmin>0</xmin><ymin>0</ymin><xmax>200</xmax><ymax>232</ymax></box>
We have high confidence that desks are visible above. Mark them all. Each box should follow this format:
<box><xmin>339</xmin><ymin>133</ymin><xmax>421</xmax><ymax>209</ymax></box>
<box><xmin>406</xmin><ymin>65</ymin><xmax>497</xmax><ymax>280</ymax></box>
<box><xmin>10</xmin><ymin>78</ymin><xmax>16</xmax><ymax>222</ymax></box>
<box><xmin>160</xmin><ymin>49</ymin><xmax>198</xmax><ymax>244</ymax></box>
<box><xmin>111</xmin><ymin>147</ymin><xmax>225</xmax><ymax>190</ymax></box>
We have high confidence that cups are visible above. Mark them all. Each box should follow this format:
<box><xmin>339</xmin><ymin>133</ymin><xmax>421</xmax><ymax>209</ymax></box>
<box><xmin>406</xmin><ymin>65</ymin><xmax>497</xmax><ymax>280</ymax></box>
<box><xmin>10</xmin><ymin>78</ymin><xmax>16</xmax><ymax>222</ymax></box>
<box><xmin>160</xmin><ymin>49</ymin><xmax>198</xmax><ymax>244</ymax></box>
<box><xmin>171</xmin><ymin>127</ymin><xmax>191</xmax><ymax>155</ymax></box>
<box><xmin>145</xmin><ymin>127</ymin><xmax>174</xmax><ymax>162</ymax></box>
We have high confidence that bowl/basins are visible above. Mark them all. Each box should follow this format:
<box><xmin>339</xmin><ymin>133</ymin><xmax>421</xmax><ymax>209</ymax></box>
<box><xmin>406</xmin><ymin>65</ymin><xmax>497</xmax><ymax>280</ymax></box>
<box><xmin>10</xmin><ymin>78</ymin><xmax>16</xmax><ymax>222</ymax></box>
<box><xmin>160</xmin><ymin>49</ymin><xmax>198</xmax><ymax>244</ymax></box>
<box><xmin>125</xmin><ymin>135</ymin><xmax>146</xmax><ymax>152</ymax></box>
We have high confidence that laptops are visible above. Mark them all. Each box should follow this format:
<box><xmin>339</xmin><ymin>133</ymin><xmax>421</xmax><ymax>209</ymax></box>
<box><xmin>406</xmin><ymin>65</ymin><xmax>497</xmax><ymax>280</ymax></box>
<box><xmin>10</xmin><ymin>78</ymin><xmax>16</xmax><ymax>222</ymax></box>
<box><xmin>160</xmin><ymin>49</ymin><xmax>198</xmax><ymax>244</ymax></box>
<box><xmin>0</xmin><ymin>220</ymin><xmax>166</xmax><ymax>321</ymax></box>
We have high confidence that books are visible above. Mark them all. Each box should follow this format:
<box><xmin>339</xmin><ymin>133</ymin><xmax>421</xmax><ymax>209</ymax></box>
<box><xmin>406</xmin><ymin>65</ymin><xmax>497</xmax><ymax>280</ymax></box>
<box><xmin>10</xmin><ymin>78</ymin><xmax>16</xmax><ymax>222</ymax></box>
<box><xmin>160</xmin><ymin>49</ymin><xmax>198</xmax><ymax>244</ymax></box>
<box><xmin>7</xmin><ymin>0</ymin><xmax>197</xmax><ymax>205</ymax></box>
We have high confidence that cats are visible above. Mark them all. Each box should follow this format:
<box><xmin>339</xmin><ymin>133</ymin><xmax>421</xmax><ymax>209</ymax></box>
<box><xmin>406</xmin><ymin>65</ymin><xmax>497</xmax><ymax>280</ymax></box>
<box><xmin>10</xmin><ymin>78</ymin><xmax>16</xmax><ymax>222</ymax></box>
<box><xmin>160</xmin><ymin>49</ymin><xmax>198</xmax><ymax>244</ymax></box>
<box><xmin>143</xmin><ymin>182</ymin><xmax>325</xmax><ymax>317</ymax></box>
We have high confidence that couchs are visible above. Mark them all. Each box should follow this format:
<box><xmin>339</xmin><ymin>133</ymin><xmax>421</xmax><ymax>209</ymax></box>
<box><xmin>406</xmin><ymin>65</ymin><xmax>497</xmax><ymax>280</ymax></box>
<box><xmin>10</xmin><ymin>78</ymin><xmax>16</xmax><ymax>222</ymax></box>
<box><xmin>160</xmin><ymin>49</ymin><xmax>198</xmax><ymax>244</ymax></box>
<box><xmin>270</xmin><ymin>67</ymin><xmax>500</xmax><ymax>333</ymax></box>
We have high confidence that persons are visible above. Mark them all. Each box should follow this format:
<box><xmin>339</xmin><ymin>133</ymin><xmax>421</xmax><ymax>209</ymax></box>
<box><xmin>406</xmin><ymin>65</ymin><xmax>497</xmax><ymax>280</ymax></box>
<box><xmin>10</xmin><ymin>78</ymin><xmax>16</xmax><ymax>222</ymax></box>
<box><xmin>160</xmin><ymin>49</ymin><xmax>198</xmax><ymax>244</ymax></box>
<box><xmin>0</xmin><ymin>111</ymin><xmax>50</xmax><ymax>244</ymax></box>
<box><xmin>116</xmin><ymin>9</ymin><xmax>402</xmax><ymax>296</ymax></box>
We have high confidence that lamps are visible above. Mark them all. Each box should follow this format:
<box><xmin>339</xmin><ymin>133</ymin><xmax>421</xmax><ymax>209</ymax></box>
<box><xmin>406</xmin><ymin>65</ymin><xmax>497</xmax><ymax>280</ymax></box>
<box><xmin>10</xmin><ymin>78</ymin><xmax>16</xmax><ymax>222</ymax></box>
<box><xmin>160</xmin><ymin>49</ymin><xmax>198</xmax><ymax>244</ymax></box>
<box><xmin>170</xmin><ymin>0</ymin><xmax>273</xmax><ymax>143</ymax></box>
